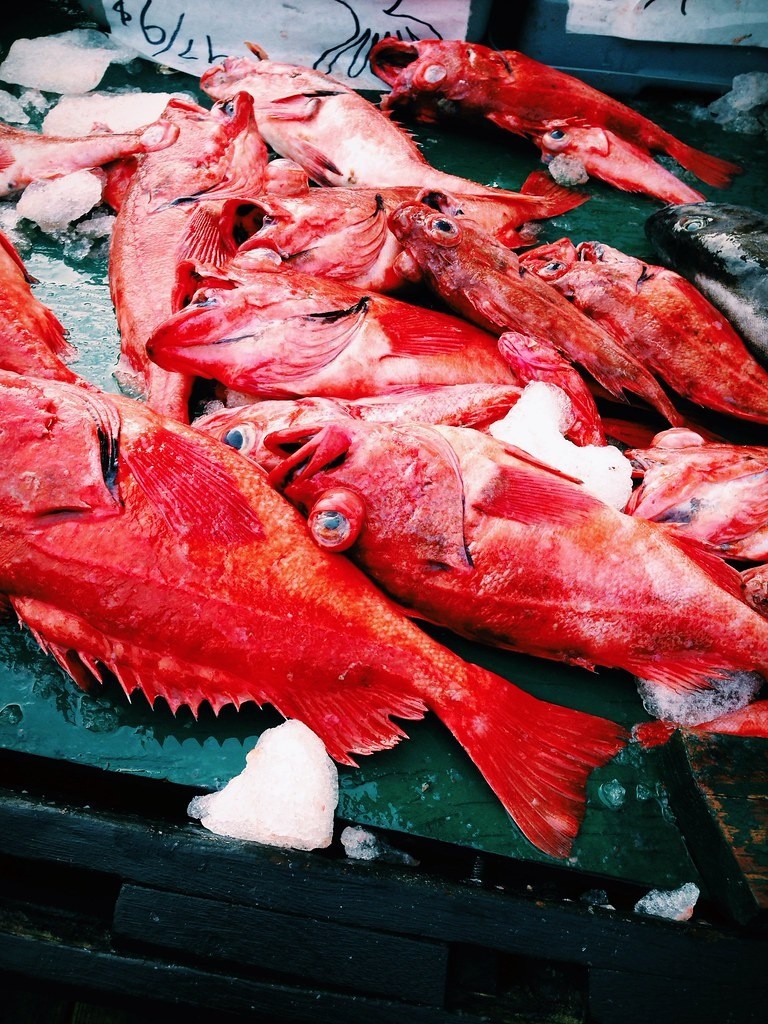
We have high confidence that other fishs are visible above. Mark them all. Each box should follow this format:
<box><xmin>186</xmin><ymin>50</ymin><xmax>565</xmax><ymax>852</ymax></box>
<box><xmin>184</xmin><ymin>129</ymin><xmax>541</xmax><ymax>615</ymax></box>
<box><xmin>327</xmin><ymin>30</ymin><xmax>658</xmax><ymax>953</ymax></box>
<box><xmin>0</xmin><ymin>38</ymin><xmax>768</xmax><ymax>859</ymax></box>
